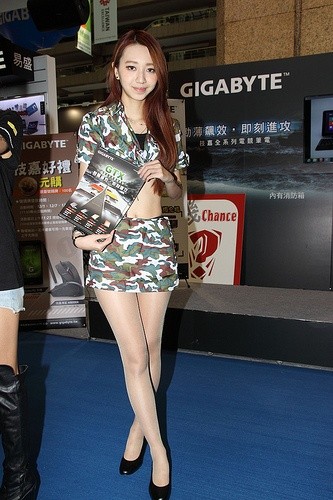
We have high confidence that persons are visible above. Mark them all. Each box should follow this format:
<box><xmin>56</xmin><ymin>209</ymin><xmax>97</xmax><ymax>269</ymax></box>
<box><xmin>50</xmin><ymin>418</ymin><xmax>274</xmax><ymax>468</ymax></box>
<box><xmin>72</xmin><ymin>31</ymin><xmax>188</xmax><ymax>500</ymax></box>
<box><xmin>1</xmin><ymin>109</ymin><xmax>43</xmax><ymax>500</ymax></box>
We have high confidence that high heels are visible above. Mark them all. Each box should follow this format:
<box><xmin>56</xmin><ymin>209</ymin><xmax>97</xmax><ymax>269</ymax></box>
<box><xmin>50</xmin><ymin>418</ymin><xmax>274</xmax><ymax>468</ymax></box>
<box><xmin>118</xmin><ymin>433</ymin><xmax>146</xmax><ymax>475</ymax></box>
<box><xmin>148</xmin><ymin>459</ymin><xmax>173</xmax><ymax>500</ymax></box>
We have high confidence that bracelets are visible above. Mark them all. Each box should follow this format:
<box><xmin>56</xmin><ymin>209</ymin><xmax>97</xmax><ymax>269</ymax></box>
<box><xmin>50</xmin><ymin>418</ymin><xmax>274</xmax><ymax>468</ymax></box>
<box><xmin>73</xmin><ymin>236</ymin><xmax>84</xmax><ymax>248</ymax></box>
<box><xmin>72</xmin><ymin>227</ymin><xmax>84</xmax><ymax>239</ymax></box>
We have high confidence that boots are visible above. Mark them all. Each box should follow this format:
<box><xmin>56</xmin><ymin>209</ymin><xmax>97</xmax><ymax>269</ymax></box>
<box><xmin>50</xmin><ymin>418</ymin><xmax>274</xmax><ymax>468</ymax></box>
<box><xmin>0</xmin><ymin>363</ymin><xmax>38</xmax><ymax>500</ymax></box>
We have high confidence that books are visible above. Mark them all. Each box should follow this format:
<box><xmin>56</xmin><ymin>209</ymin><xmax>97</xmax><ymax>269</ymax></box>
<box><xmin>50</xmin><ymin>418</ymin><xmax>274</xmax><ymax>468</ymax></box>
<box><xmin>56</xmin><ymin>145</ymin><xmax>148</xmax><ymax>241</ymax></box>
